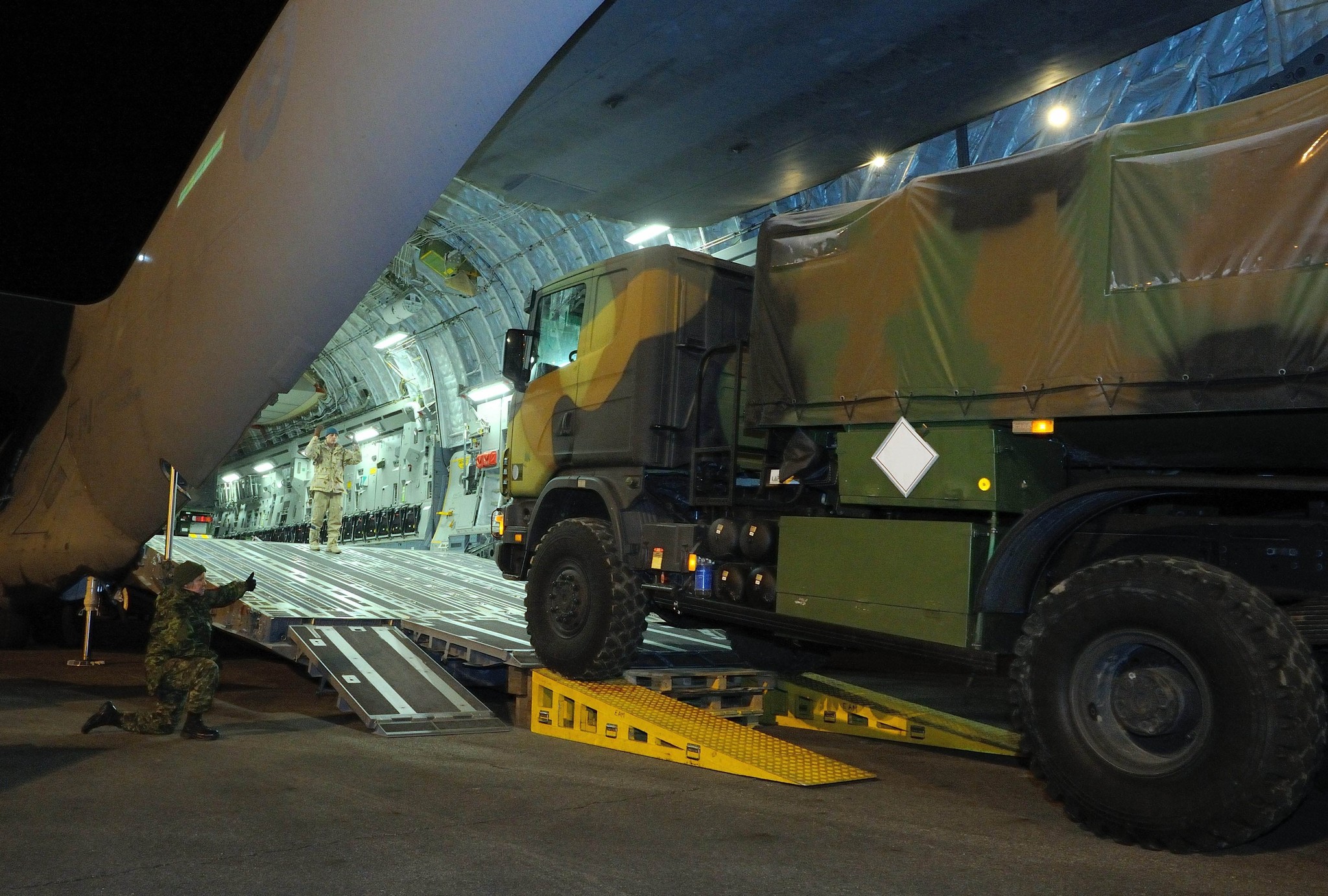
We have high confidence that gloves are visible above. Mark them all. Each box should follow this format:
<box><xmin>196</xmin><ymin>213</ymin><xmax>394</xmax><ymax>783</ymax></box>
<box><xmin>349</xmin><ymin>431</ymin><xmax>358</xmax><ymax>443</ymax></box>
<box><xmin>246</xmin><ymin>572</ymin><xmax>256</xmax><ymax>592</ymax></box>
<box><xmin>314</xmin><ymin>425</ymin><xmax>324</xmax><ymax>437</ymax></box>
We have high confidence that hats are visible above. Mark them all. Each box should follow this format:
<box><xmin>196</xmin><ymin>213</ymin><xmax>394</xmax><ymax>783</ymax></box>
<box><xmin>325</xmin><ymin>427</ymin><xmax>338</xmax><ymax>436</ymax></box>
<box><xmin>174</xmin><ymin>561</ymin><xmax>207</xmax><ymax>587</ymax></box>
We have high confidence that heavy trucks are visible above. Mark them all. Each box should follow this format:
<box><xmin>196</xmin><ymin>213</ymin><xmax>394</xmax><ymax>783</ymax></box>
<box><xmin>491</xmin><ymin>72</ymin><xmax>1326</xmax><ymax>854</ymax></box>
<box><xmin>174</xmin><ymin>510</ymin><xmax>216</xmax><ymax>539</ymax></box>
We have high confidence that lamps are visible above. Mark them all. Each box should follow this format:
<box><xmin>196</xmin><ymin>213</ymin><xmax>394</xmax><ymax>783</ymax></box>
<box><xmin>622</xmin><ymin>223</ymin><xmax>670</xmax><ymax>244</ymax></box>
<box><xmin>251</xmin><ymin>458</ymin><xmax>277</xmax><ymax>472</ymax></box>
<box><xmin>349</xmin><ymin>426</ymin><xmax>379</xmax><ymax>442</ymax></box>
<box><xmin>220</xmin><ymin>470</ymin><xmax>242</xmax><ymax>483</ymax></box>
<box><xmin>467</xmin><ymin>380</ymin><xmax>514</xmax><ymax>401</ymax></box>
<box><xmin>373</xmin><ymin>331</ymin><xmax>409</xmax><ymax>349</ymax></box>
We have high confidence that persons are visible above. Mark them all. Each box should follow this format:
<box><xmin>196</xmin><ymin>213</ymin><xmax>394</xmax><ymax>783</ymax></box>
<box><xmin>81</xmin><ymin>561</ymin><xmax>257</xmax><ymax>740</ymax></box>
<box><xmin>304</xmin><ymin>425</ymin><xmax>363</xmax><ymax>553</ymax></box>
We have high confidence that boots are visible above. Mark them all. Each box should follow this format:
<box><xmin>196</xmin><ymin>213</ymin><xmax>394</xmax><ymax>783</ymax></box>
<box><xmin>310</xmin><ymin>540</ymin><xmax>320</xmax><ymax>550</ymax></box>
<box><xmin>181</xmin><ymin>719</ymin><xmax>219</xmax><ymax>741</ymax></box>
<box><xmin>326</xmin><ymin>540</ymin><xmax>342</xmax><ymax>553</ymax></box>
<box><xmin>82</xmin><ymin>701</ymin><xmax>120</xmax><ymax>733</ymax></box>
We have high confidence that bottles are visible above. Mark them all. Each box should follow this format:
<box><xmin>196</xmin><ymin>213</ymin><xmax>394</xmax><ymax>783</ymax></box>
<box><xmin>694</xmin><ymin>558</ymin><xmax>712</xmax><ymax>598</ymax></box>
